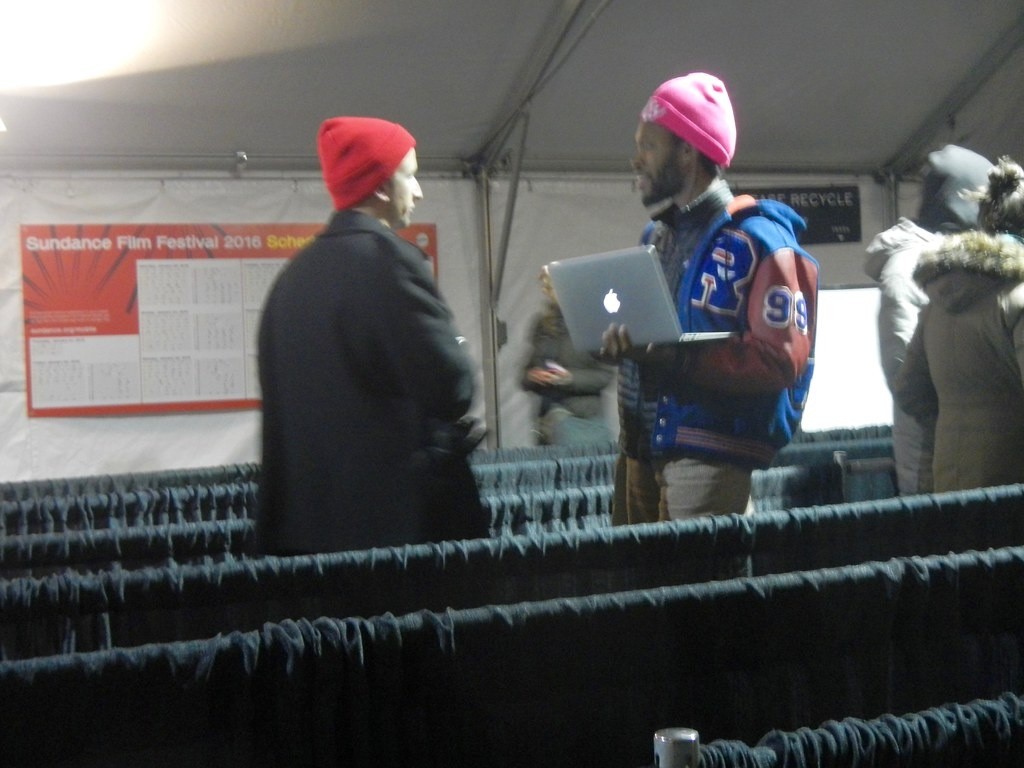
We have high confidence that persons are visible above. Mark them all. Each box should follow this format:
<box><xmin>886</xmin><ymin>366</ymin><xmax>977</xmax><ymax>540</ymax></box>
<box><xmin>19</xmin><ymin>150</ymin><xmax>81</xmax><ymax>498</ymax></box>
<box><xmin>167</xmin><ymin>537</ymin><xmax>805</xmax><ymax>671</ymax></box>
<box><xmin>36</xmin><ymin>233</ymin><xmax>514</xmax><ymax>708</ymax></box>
<box><xmin>865</xmin><ymin>145</ymin><xmax>1024</xmax><ymax>498</ymax></box>
<box><xmin>520</xmin><ymin>71</ymin><xmax>818</xmax><ymax>524</ymax></box>
<box><xmin>257</xmin><ymin>118</ymin><xmax>488</xmax><ymax>557</ymax></box>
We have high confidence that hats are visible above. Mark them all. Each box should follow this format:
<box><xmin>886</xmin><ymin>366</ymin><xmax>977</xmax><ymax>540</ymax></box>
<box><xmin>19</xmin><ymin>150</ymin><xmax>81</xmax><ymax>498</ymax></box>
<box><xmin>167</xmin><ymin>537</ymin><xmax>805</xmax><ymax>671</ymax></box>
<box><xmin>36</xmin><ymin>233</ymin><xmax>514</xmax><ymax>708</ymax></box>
<box><xmin>318</xmin><ymin>117</ymin><xmax>415</xmax><ymax>210</ymax></box>
<box><xmin>641</xmin><ymin>73</ymin><xmax>737</xmax><ymax>166</ymax></box>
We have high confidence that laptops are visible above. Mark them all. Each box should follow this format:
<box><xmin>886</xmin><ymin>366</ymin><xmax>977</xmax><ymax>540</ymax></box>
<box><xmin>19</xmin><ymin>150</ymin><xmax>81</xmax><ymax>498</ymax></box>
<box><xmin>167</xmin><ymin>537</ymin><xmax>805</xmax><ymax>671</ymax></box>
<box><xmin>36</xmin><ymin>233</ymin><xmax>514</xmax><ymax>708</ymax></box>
<box><xmin>549</xmin><ymin>243</ymin><xmax>741</xmax><ymax>354</ymax></box>
